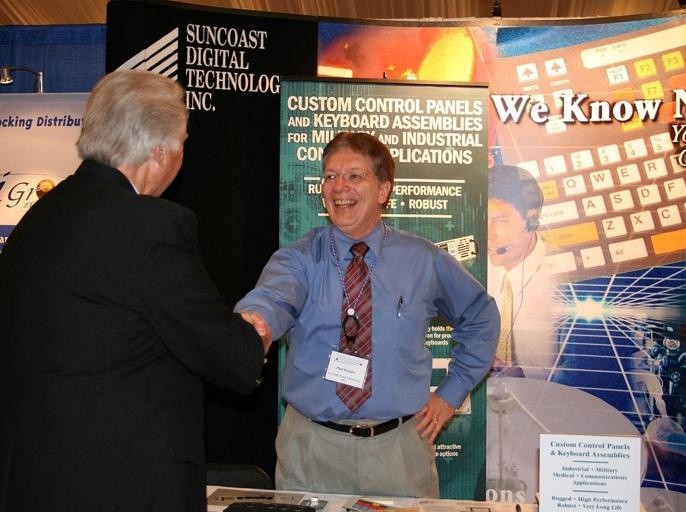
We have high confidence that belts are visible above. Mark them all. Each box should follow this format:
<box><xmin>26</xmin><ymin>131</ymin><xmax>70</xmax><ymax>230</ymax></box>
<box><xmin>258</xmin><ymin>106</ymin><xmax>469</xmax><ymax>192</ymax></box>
<box><xmin>313</xmin><ymin>415</ymin><xmax>416</xmax><ymax>438</ymax></box>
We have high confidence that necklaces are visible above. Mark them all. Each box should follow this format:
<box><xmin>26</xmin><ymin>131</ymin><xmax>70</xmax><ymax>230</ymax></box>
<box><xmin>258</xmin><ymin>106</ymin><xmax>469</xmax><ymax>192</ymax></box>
<box><xmin>329</xmin><ymin>222</ymin><xmax>388</xmax><ymax>344</ymax></box>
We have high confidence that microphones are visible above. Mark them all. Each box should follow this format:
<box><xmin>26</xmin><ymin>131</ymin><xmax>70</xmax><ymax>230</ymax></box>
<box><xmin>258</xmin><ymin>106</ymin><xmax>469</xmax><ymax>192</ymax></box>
<box><xmin>497</xmin><ymin>216</ymin><xmax>539</xmax><ymax>255</ymax></box>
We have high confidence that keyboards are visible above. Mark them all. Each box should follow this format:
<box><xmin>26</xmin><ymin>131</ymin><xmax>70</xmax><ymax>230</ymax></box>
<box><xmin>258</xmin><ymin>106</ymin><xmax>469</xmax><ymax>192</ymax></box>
<box><xmin>489</xmin><ymin>16</ymin><xmax>686</xmax><ymax>285</ymax></box>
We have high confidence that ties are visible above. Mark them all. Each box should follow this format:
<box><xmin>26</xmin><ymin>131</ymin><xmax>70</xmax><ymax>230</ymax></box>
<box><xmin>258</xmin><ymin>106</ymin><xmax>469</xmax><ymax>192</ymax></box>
<box><xmin>335</xmin><ymin>241</ymin><xmax>373</xmax><ymax>413</ymax></box>
<box><xmin>496</xmin><ymin>275</ymin><xmax>514</xmax><ymax>369</ymax></box>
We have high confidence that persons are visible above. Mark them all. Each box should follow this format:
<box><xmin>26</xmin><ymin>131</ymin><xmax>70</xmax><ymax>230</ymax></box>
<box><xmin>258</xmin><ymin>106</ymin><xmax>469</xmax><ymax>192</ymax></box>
<box><xmin>0</xmin><ymin>69</ymin><xmax>272</xmax><ymax>512</ymax></box>
<box><xmin>233</xmin><ymin>132</ymin><xmax>501</xmax><ymax>498</ymax></box>
<box><xmin>487</xmin><ymin>165</ymin><xmax>558</xmax><ymax>379</ymax></box>
